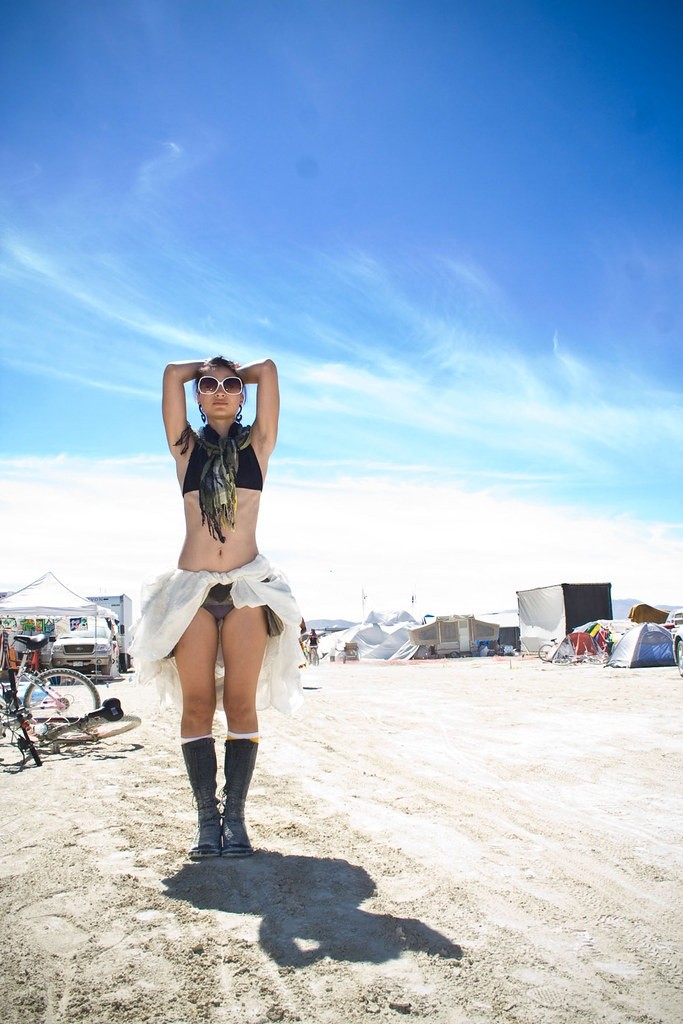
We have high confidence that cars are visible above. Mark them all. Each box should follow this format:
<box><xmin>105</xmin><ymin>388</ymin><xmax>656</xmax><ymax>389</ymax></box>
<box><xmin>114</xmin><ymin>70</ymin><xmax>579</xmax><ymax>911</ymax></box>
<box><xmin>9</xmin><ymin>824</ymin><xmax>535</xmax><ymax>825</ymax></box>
<box><xmin>672</xmin><ymin>625</ymin><xmax>683</xmax><ymax>678</ymax></box>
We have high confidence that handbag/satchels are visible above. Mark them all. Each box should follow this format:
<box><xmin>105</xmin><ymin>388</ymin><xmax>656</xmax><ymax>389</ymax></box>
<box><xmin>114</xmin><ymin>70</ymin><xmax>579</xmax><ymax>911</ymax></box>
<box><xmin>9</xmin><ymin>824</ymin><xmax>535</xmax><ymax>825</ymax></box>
<box><xmin>265</xmin><ymin>605</ymin><xmax>284</xmax><ymax>638</ymax></box>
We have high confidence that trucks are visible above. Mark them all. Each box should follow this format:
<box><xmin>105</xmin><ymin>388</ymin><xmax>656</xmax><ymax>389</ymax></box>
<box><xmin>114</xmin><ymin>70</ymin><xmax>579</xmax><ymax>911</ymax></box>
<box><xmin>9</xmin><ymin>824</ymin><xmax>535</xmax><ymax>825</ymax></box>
<box><xmin>48</xmin><ymin>592</ymin><xmax>133</xmax><ymax>674</ymax></box>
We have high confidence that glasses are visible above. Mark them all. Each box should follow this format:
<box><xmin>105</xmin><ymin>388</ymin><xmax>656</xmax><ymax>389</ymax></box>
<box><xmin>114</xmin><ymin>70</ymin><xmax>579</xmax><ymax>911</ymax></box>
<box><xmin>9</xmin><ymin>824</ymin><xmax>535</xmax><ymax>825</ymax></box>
<box><xmin>196</xmin><ymin>376</ymin><xmax>244</xmax><ymax>395</ymax></box>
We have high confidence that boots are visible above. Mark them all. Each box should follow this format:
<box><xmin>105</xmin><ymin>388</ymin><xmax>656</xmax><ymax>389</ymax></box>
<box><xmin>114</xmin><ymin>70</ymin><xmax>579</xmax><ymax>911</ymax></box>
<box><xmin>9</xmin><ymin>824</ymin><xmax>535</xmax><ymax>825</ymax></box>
<box><xmin>221</xmin><ymin>739</ymin><xmax>259</xmax><ymax>857</ymax></box>
<box><xmin>182</xmin><ymin>737</ymin><xmax>221</xmax><ymax>857</ymax></box>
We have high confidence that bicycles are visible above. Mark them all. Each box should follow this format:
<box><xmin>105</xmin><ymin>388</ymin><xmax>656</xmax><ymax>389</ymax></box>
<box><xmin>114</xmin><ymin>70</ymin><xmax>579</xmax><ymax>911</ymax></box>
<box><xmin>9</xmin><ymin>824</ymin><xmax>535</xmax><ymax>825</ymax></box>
<box><xmin>539</xmin><ymin>637</ymin><xmax>558</xmax><ymax>662</ymax></box>
<box><xmin>0</xmin><ymin>633</ymin><xmax>100</xmax><ymax>738</ymax></box>
<box><xmin>305</xmin><ymin>641</ymin><xmax>320</xmax><ymax>667</ymax></box>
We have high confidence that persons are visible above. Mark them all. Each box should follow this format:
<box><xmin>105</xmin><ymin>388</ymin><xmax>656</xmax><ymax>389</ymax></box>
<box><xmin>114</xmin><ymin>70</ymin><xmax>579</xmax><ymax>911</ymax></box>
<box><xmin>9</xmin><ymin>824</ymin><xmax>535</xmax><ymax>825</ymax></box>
<box><xmin>304</xmin><ymin>629</ymin><xmax>319</xmax><ymax>665</ymax></box>
<box><xmin>128</xmin><ymin>355</ymin><xmax>307</xmax><ymax>859</ymax></box>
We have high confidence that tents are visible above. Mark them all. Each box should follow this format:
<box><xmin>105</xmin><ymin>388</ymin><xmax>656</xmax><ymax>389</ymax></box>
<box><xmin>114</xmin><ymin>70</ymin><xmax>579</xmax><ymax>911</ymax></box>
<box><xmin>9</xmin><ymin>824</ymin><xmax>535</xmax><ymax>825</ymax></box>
<box><xmin>551</xmin><ymin>619</ymin><xmax>674</xmax><ymax>670</ymax></box>
<box><xmin>0</xmin><ymin>572</ymin><xmax>118</xmax><ymax>684</ymax></box>
